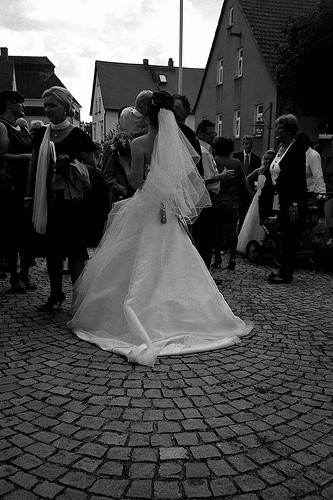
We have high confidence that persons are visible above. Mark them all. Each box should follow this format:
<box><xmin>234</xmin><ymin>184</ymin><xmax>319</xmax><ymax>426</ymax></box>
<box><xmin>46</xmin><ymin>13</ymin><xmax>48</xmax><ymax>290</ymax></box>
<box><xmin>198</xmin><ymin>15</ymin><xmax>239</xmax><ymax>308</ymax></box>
<box><xmin>233</xmin><ymin>136</ymin><xmax>261</xmax><ymax>178</ymax></box>
<box><xmin>67</xmin><ymin>91</ymin><xmax>254</xmax><ymax>367</ymax></box>
<box><xmin>26</xmin><ymin>85</ymin><xmax>97</xmax><ymax>315</ymax></box>
<box><xmin>99</xmin><ymin>106</ymin><xmax>137</xmax><ymax>207</ymax></box>
<box><xmin>298</xmin><ymin>133</ymin><xmax>327</xmax><ymax>200</ymax></box>
<box><xmin>237</xmin><ymin>150</ymin><xmax>279</xmax><ymax>255</ymax></box>
<box><xmin>192</xmin><ymin>120</ymin><xmax>234</xmax><ymax>284</ymax></box>
<box><xmin>211</xmin><ymin>137</ymin><xmax>251</xmax><ymax>270</ymax></box>
<box><xmin>0</xmin><ymin>90</ymin><xmax>44</xmax><ymax>295</ymax></box>
<box><xmin>173</xmin><ymin>93</ymin><xmax>204</xmax><ymax>176</ymax></box>
<box><xmin>264</xmin><ymin>114</ymin><xmax>309</xmax><ymax>284</ymax></box>
<box><xmin>90</xmin><ymin>142</ymin><xmax>109</xmax><ymax>243</ymax></box>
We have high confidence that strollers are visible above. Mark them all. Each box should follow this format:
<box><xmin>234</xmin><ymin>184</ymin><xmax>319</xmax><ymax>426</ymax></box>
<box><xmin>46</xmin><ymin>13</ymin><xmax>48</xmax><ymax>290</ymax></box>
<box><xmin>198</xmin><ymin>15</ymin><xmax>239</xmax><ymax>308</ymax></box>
<box><xmin>245</xmin><ymin>206</ymin><xmax>322</xmax><ymax>267</ymax></box>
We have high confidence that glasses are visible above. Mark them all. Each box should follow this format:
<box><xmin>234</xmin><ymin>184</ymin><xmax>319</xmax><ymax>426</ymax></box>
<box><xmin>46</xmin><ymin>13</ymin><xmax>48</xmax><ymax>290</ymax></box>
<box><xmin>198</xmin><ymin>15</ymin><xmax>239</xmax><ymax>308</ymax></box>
<box><xmin>200</xmin><ymin>129</ymin><xmax>218</xmax><ymax>137</ymax></box>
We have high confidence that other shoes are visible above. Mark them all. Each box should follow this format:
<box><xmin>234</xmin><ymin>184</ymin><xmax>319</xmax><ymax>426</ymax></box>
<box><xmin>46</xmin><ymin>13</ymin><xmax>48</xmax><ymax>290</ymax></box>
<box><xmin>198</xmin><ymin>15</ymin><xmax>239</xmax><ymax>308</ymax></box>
<box><xmin>18</xmin><ymin>274</ymin><xmax>37</xmax><ymax>291</ymax></box>
<box><xmin>268</xmin><ymin>273</ymin><xmax>292</xmax><ymax>284</ymax></box>
<box><xmin>9</xmin><ymin>278</ymin><xmax>27</xmax><ymax>294</ymax></box>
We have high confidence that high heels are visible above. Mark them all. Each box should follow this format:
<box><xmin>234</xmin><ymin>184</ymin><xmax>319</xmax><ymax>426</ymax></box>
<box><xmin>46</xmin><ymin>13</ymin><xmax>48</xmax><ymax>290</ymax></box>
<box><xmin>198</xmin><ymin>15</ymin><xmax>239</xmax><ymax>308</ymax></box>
<box><xmin>211</xmin><ymin>257</ymin><xmax>223</xmax><ymax>269</ymax></box>
<box><xmin>227</xmin><ymin>261</ymin><xmax>236</xmax><ymax>271</ymax></box>
<box><xmin>40</xmin><ymin>292</ymin><xmax>66</xmax><ymax>311</ymax></box>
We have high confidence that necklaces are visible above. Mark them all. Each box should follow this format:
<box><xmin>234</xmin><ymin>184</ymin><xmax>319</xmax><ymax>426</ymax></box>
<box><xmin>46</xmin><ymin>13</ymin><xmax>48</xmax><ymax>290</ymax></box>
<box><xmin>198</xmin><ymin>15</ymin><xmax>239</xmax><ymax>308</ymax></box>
<box><xmin>55</xmin><ymin>135</ymin><xmax>57</xmax><ymax>137</ymax></box>
